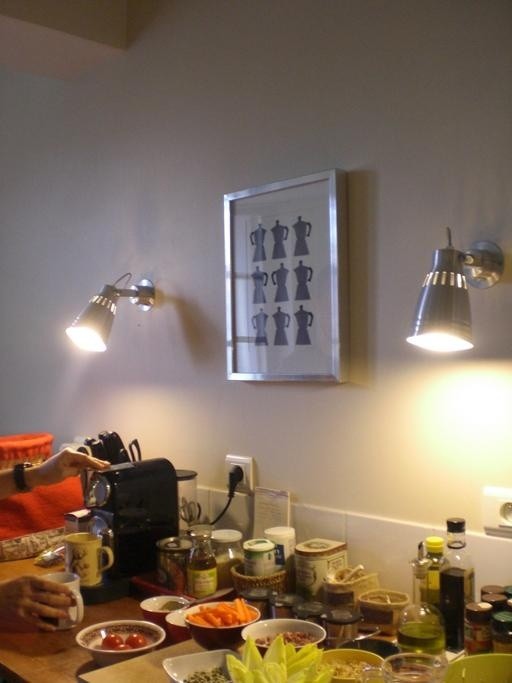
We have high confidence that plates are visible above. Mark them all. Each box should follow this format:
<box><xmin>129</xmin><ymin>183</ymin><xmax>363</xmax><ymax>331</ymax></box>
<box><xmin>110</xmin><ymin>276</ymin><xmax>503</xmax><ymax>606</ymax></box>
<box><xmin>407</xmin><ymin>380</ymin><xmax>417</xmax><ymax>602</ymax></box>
<box><xmin>129</xmin><ymin>573</ymin><xmax>234</xmax><ymax>606</ymax></box>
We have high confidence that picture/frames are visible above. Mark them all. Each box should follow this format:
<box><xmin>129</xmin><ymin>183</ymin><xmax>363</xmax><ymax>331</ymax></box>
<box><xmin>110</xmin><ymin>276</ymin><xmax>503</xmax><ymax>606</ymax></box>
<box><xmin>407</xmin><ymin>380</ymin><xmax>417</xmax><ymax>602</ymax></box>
<box><xmin>224</xmin><ymin>168</ymin><xmax>349</xmax><ymax>384</ymax></box>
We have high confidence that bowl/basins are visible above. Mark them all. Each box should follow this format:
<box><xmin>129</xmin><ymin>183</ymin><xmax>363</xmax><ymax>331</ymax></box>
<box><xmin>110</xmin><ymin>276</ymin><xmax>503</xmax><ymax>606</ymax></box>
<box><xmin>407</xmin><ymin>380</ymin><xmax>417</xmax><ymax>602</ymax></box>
<box><xmin>316</xmin><ymin>648</ymin><xmax>384</xmax><ymax>683</ymax></box>
<box><xmin>140</xmin><ymin>593</ymin><xmax>189</xmax><ymax>624</ymax></box>
<box><xmin>183</xmin><ymin>600</ymin><xmax>261</xmax><ymax>652</ymax></box>
<box><xmin>163</xmin><ymin>647</ymin><xmax>241</xmax><ymax>681</ymax></box>
<box><xmin>239</xmin><ymin>619</ymin><xmax>328</xmax><ymax>651</ymax></box>
<box><xmin>75</xmin><ymin>619</ymin><xmax>167</xmax><ymax>668</ymax></box>
<box><xmin>164</xmin><ymin>607</ymin><xmax>189</xmax><ymax>640</ymax></box>
<box><xmin>384</xmin><ymin>652</ymin><xmax>445</xmax><ymax>683</ymax></box>
<box><xmin>447</xmin><ymin>652</ymin><xmax>511</xmax><ymax>682</ymax></box>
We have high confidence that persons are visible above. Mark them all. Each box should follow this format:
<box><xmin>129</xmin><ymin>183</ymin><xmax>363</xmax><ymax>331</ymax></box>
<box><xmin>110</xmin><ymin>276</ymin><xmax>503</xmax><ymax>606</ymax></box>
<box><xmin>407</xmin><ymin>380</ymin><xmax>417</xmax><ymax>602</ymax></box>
<box><xmin>1</xmin><ymin>449</ymin><xmax>109</xmax><ymax>633</ymax></box>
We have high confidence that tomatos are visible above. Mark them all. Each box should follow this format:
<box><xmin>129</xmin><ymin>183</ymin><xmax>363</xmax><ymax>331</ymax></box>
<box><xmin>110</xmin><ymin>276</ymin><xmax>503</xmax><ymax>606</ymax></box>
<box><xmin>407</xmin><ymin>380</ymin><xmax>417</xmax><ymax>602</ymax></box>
<box><xmin>126</xmin><ymin>633</ymin><xmax>147</xmax><ymax>646</ymax></box>
<box><xmin>101</xmin><ymin>634</ymin><xmax>123</xmax><ymax>649</ymax></box>
<box><xmin>115</xmin><ymin>644</ymin><xmax>132</xmax><ymax>649</ymax></box>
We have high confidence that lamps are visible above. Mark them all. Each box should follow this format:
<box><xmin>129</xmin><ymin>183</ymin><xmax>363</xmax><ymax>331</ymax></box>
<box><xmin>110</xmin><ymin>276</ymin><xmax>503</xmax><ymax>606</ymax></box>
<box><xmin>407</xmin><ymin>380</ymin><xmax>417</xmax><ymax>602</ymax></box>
<box><xmin>65</xmin><ymin>273</ymin><xmax>154</xmax><ymax>352</ymax></box>
<box><xmin>406</xmin><ymin>227</ymin><xmax>503</xmax><ymax>353</ymax></box>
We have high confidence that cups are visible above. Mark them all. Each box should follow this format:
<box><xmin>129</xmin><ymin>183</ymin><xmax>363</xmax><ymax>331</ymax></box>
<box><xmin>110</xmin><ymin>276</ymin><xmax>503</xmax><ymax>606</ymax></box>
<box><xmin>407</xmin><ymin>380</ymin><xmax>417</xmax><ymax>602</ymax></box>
<box><xmin>64</xmin><ymin>531</ymin><xmax>115</xmax><ymax>587</ymax></box>
<box><xmin>38</xmin><ymin>571</ymin><xmax>85</xmax><ymax>629</ymax></box>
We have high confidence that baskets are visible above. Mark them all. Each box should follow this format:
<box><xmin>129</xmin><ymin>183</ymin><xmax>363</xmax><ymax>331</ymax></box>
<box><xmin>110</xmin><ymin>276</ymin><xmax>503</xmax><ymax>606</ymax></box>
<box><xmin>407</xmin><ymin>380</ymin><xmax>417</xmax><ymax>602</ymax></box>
<box><xmin>322</xmin><ymin>569</ymin><xmax>378</xmax><ymax>610</ymax></box>
<box><xmin>230</xmin><ymin>563</ymin><xmax>289</xmax><ymax>600</ymax></box>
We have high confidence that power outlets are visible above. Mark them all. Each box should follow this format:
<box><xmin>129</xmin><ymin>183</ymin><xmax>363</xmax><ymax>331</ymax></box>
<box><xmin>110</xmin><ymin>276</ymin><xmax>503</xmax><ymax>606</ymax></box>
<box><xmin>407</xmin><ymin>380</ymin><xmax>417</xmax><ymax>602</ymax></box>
<box><xmin>224</xmin><ymin>453</ymin><xmax>253</xmax><ymax>493</ymax></box>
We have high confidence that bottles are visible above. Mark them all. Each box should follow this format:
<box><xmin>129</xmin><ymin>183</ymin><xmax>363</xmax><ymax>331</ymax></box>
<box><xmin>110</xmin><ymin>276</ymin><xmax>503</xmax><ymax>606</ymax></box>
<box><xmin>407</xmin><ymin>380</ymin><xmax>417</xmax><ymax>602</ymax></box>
<box><xmin>483</xmin><ymin>594</ymin><xmax>506</xmax><ymax>613</ymax></box>
<box><xmin>492</xmin><ymin>612</ymin><xmax>512</xmax><ymax>653</ymax></box>
<box><xmin>187</xmin><ymin>526</ymin><xmax>216</xmax><ymax>598</ymax></box>
<box><xmin>463</xmin><ymin>603</ymin><xmax>492</xmax><ymax>654</ymax></box>
<box><xmin>176</xmin><ymin>470</ymin><xmax>197</xmax><ymax>538</ymax></box>
<box><xmin>481</xmin><ymin>585</ymin><xmax>504</xmax><ymax>595</ymax></box>
<box><xmin>440</xmin><ymin>520</ymin><xmax>475</xmax><ymax>650</ymax></box>
<box><xmin>507</xmin><ymin>599</ymin><xmax>512</xmax><ymax>611</ymax></box>
<box><xmin>503</xmin><ymin>586</ymin><xmax>511</xmax><ymax>596</ymax></box>
<box><xmin>211</xmin><ymin>530</ymin><xmax>243</xmax><ymax>590</ymax></box>
<box><xmin>421</xmin><ymin>538</ymin><xmax>446</xmax><ymax>605</ymax></box>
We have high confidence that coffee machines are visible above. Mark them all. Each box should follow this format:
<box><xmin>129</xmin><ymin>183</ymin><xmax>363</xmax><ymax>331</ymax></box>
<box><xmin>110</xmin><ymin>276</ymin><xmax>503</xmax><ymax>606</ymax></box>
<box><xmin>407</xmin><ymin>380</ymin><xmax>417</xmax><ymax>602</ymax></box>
<box><xmin>63</xmin><ymin>459</ymin><xmax>179</xmax><ymax>608</ymax></box>
